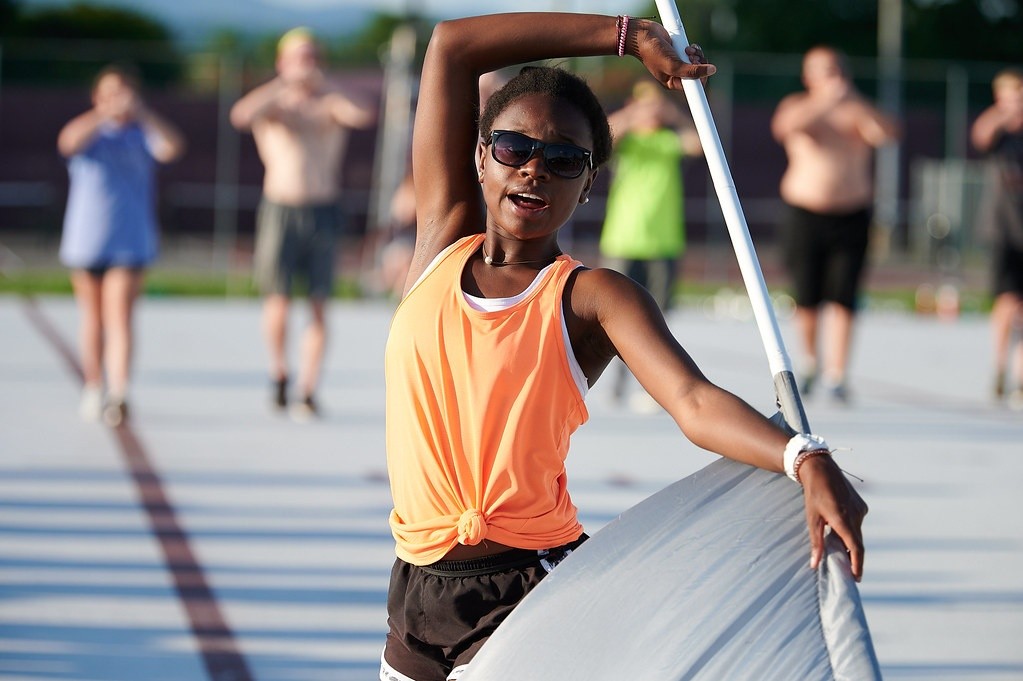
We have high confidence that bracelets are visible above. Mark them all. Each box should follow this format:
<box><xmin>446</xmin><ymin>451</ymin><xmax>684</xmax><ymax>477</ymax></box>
<box><xmin>794</xmin><ymin>450</ymin><xmax>830</xmax><ymax>482</ymax></box>
<box><xmin>784</xmin><ymin>433</ymin><xmax>829</xmax><ymax>481</ymax></box>
<box><xmin>619</xmin><ymin>15</ymin><xmax>628</xmax><ymax>56</ymax></box>
<box><xmin>616</xmin><ymin>16</ymin><xmax>621</xmax><ymax>52</ymax></box>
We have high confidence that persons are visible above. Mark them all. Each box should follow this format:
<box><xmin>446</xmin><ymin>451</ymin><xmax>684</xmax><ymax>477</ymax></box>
<box><xmin>772</xmin><ymin>49</ymin><xmax>890</xmax><ymax>409</ymax></box>
<box><xmin>382</xmin><ymin>59</ymin><xmax>504</xmax><ymax>297</ymax></box>
<box><xmin>597</xmin><ymin>81</ymin><xmax>701</xmax><ymax>411</ymax></box>
<box><xmin>57</xmin><ymin>68</ymin><xmax>179</xmax><ymax>423</ymax></box>
<box><xmin>972</xmin><ymin>73</ymin><xmax>1023</xmax><ymax>404</ymax></box>
<box><xmin>230</xmin><ymin>27</ymin><xmax>376</xmax><ymax>415</ymax></box>
<box><xmin>380</xmin><ymin>12</ymin><xmax>867</xmax><ymax>680</ymax></box>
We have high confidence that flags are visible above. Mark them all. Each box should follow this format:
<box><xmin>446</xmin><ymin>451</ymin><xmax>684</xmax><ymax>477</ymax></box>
<box><xmin>458</xmin><ymin>412</ymin><xmax>882</xmax><ymax>681</ymax></box>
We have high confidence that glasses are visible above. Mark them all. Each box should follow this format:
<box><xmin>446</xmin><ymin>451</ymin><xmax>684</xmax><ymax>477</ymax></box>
<box><xmin>484</xmin><ymin>130</ymin><xmax>593</xmax><ymax>179</ymax></box>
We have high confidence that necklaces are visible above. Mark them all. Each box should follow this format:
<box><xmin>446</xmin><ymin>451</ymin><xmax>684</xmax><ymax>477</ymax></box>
<box><xmin>483</xmin><ymin>245</ymin><xmax>562</xmax><ymax>265</ymax></box>
